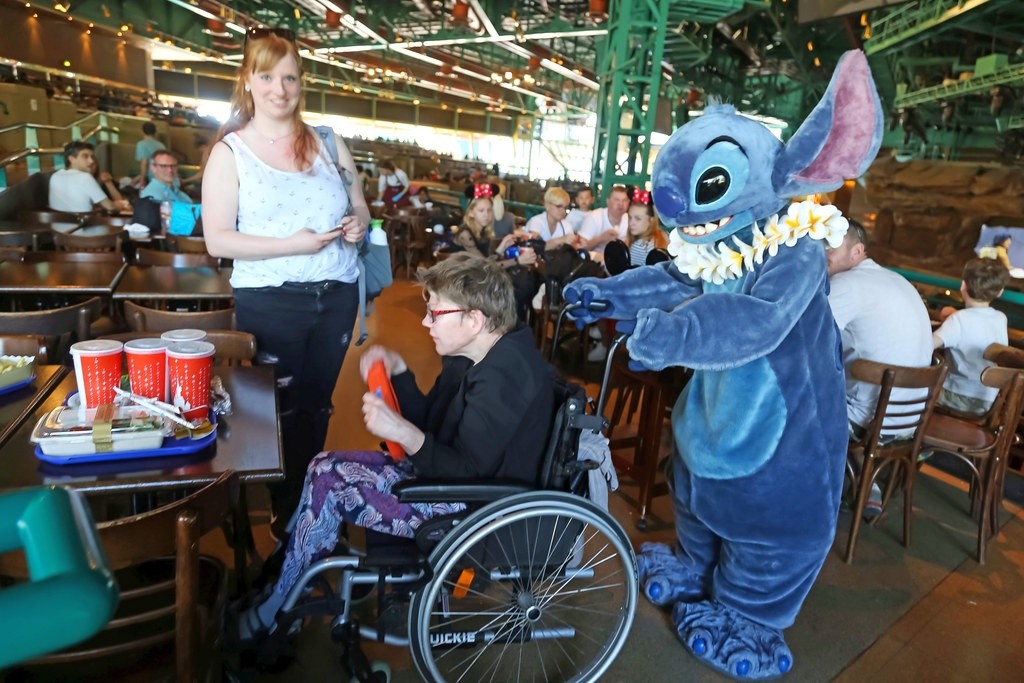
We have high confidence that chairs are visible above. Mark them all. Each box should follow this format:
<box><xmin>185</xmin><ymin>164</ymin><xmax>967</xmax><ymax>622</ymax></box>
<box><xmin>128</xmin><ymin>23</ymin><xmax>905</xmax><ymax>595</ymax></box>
<box><xmin>0</xmin><ymin>206</ymin><xmax>266</xmax><ymax>683</ymax></box>
<box><xmin>367</xmin><ymin>191</ymin><xmax>1024</xmax><ymax>569</ymax></box>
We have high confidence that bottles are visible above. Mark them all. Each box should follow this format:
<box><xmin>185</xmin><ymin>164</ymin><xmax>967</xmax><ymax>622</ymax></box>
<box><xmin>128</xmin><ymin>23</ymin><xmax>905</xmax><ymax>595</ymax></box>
<box><xmin>367</xmin><ymin>218</ymin><xmax>388</xmax><ymax>246</ymax></box>
<box><xmin>159</xmin><ymin>191</ymin><xmax>171</xmax><ymax>236</ymax></box>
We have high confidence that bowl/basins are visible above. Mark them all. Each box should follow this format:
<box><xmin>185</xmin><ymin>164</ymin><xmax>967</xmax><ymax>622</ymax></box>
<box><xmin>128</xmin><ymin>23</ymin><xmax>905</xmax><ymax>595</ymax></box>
<box><xmin>30</xmin><ymin>405</ymin><xmax>175</xmax><ymax>457</ymax></box>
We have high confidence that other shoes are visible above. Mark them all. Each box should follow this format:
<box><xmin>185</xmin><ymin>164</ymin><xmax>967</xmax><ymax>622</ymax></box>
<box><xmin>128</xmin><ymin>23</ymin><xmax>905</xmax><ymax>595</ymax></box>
<box><xmin>863</xmin><ymin>488</ymin><xmax>882</xmax><ymax>517</ymax></box>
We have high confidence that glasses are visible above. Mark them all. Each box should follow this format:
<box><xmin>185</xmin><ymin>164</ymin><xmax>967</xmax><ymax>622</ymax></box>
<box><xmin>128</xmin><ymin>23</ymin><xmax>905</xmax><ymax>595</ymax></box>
<box><xmin>846</xmin><ymin>216</ymin><xmax>862</xmax><ymax>242</ymax></box>
<box><xmin>548</xmin><ymin>202</ymin><xmax>568</xmax><ymax>209</ymax></box>
<box><xmin>244</xmin><ymin>28</ymin><xmax>295</xmax><ymax>58</ymax></box>
<box><xmin>154</xmin><ymin>163</ymin><xmax>178</xmax><ymax>169</ymax></box>
<box><xmin>426</xmin><ymin>303</ymin><xmax>466</xmax><ymax>324</ymax></box>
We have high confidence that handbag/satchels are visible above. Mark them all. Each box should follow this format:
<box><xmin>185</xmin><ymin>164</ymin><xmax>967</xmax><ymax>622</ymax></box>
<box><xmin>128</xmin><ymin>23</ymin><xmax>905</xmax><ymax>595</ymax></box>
<box><xmin>313</xmin><ymin>125</ymin><xmax>392</xmax><ymax>302</ymax></box>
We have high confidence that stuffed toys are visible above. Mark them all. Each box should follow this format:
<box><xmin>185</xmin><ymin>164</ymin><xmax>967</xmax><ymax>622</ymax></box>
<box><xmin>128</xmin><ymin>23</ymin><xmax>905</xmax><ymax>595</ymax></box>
<box><xmin>562</xmin><ymin>49</ymin><xmax>884</xmax><ymax>679</ymax></box>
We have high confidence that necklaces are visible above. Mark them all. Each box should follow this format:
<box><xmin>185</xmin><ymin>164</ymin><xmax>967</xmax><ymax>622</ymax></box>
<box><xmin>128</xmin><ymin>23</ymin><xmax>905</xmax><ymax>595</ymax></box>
<box><xmin>250</xmin><ymin>116</ymin><xmax>292</xmax><ymax>145</ymax></box>
<box><xmin>492</xmin><ymin>335</ymin><xmax>501</xmax><ymax>347</ymax></box>
<box><xmin>668</xmin><ymin>200</ymin><xmax>850</xmax><ymax>286</ymax></box>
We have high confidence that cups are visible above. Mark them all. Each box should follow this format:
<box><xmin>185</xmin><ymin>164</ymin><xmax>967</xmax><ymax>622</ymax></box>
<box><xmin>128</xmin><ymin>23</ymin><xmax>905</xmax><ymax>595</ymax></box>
<box><xmin>165</xmin><ymin>339</ymin><xmax>216</xmax><ymax>416</ymax></box>
<box><xmin>123</xmin><ymin>338</ymin><xmax>170</xmax><ymax>403</ymax></box>
<box><xmin>161</xmin><ymin>329</ymin><xmax>209</xmax><ymax>341</ymax></box>
<box><xmin>69</xmin><ymin>339</ymin><xmax>124</xmax><ymax>409</ymax></box>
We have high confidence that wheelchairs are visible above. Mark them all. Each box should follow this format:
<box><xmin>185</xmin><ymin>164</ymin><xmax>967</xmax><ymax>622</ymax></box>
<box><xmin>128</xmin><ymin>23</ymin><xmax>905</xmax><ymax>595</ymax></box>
<box><xmin>206</xmin><ymin>299</ymin><xmax>640</xmax><ymax>683</ymax></box>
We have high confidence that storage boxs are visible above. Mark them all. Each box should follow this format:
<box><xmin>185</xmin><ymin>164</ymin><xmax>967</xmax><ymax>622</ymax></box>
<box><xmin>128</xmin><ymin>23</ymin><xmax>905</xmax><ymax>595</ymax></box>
<box><xmin>28</xmin><ymin>403</ymin><xmax>175</xmax><ymax>456</ymax></box>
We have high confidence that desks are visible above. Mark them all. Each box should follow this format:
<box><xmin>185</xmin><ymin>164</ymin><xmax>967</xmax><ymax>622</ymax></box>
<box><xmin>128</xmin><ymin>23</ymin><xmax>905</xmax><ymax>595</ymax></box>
<box><xmin>0</xmin><ymin>367</ymin><xmax>287</xmax><ymax>683</ymax></box>
<box><xmin>0</xmin><ymin>258</ymin><xmax>129</xmax><ymax>312</ymax></box>
<box><xmin>0</xmin><ymin>364</ymin><xmax>68</xmax><ymax>448</ymax></box>
<box><xmin>112</xmin><ymin>264</ymin><xmax>235</xmax><ymax>311</ymax></box>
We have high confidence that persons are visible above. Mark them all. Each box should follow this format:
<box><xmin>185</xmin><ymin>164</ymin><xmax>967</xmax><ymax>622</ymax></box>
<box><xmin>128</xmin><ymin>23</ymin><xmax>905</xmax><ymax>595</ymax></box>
<box><xmin>977</xmin><ymin>233</ymin><xmax>1024</xmax><ymax>279</ymax></box>
<box><xmin>825</xmin><ymin>220</ymin><xmax>934</xmax><ymax>516</ymax></box>
<box><xmin>48</xmin><ymin>122</ymin><xmax>193</xmax><ymax>237</ymax></box>
<box><xmin>355</xmin><ymin>159</ymin><xmax>671</xmax><ymax>362</ymax></box>
<box><xmin>200</xmin><ymin>25</ymin><xmax>371</xmax><ymax>543</ymax></box>
<box><xmin>917</xmin><ymin>256</ymin><xmax>1009</xmax><ymax>463</ymax></box>
<box><xmin>235</xmin><ymin>253</ymin><xmax>555</xmax><ymax>643</ymax></box>
